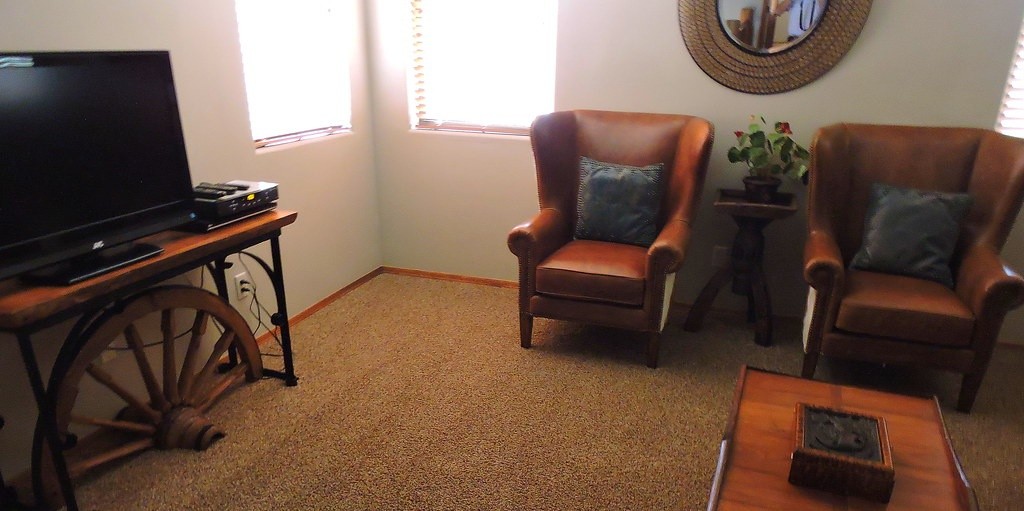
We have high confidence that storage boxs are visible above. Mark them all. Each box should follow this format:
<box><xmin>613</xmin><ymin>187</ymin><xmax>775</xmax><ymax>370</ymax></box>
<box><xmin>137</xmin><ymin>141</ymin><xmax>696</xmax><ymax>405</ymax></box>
<box><xmin>786</xmin><ymin>402</ymin><xmax>895</xmax><ymax>499</ymax></box>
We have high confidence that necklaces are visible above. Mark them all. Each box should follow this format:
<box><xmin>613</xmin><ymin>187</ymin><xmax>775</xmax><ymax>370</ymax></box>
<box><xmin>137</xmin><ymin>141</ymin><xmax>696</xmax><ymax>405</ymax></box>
<box><xmin>799</xmin><ymin>0</ymin><xmax>816</xmax><ymax>31</ymax></box>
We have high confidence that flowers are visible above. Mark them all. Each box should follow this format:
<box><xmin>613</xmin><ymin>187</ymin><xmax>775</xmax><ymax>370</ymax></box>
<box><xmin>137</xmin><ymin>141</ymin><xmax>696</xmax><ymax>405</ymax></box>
<box><xmin>727</xmin><ymin>117</ymin><xmax>810</xmax><ymax>178</ymax></box>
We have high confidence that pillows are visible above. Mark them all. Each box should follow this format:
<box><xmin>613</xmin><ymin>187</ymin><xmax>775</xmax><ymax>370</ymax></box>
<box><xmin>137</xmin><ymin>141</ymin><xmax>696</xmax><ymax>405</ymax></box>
<box><xmin>566</xmin><ymin>154</ymin><xmax>666</xmax><ymax>246</ymax></box>
<box><xmin>852</xmin><ymin>181</ymin><xmax>973</xmax><ymax>290</ymax></box>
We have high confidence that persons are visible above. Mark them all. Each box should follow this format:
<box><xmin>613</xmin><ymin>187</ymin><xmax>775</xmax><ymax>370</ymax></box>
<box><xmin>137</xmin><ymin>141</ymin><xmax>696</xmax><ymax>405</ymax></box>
<box><xmin>726</xmin><ymin>7</ymin><xmax>753</xmax><ymax>46</ymax></box>
<box><xmin>770</xmin><ymin>0</ymin><xmax>827</xmax><ymax>43</ymax></box>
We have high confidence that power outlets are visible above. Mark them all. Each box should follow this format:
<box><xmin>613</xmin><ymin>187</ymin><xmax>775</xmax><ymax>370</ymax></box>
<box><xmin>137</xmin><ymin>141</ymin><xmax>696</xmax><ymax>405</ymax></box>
<box><xmin>235</xmin><ymin>271</ymin><xmax>256</xmax><ymax>301</ymax></box>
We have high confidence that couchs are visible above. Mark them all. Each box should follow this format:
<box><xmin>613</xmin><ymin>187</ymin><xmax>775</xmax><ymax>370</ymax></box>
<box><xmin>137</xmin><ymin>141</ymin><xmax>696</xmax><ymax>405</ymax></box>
<box><xmin>508</xmin><ymin>108</ymin><xmax>715</xmax><ymax>369</ymax></box>
<box><xmin>800</xmin><ymin>124</ymin><xmax>1024</xmax><ymax>413</ymax></box>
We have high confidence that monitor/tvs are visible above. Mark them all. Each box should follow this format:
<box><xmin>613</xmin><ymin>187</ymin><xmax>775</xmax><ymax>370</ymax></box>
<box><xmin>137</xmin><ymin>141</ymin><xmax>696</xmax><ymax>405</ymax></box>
<box><xmin>0</xmin><ymin>50</ymin><xmax>200</xmax><ymax>289</ymax></box>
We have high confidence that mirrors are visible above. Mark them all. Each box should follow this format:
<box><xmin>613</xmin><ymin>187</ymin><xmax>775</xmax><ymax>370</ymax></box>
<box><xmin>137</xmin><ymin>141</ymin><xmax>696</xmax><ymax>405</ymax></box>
<box><xmin>712</xmin><ymin>1</ymin><xmax>827</xmax><ymax>57</ymax></box>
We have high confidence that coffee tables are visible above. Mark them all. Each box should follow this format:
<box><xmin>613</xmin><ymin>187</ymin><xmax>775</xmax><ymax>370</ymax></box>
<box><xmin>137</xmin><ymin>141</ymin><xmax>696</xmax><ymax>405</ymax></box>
<box><xmin>708</xmin><ymin>364</ymin><xmax>981</xmax><ymax>510</ymax></box>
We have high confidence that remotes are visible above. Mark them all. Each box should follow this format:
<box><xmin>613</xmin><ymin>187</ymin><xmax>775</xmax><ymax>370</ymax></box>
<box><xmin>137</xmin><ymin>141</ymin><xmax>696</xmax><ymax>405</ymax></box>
<box><xmin>193</xmin><ymin>181</ymin><xmax>238</xmax><ymax>200</ymax></box>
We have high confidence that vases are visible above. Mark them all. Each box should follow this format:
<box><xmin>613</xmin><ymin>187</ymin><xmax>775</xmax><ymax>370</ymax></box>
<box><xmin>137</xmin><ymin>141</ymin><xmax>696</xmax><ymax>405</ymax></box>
<box><xmin>743</xmin><ymin>172</ymin><xmax>782</xmax><ymax>199</ymax></box>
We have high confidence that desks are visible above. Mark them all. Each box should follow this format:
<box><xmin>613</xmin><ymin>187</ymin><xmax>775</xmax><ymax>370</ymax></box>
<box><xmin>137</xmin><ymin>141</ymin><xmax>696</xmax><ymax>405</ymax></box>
<box><xmin>686</xmin><ymin>190</ymin><xmax>799</xmax><ymax>344</ymax></box>
<box><xmin>0</xmin><ymin>202</ymin><xmax>299</xmax><ymax>510</ymax></box>
